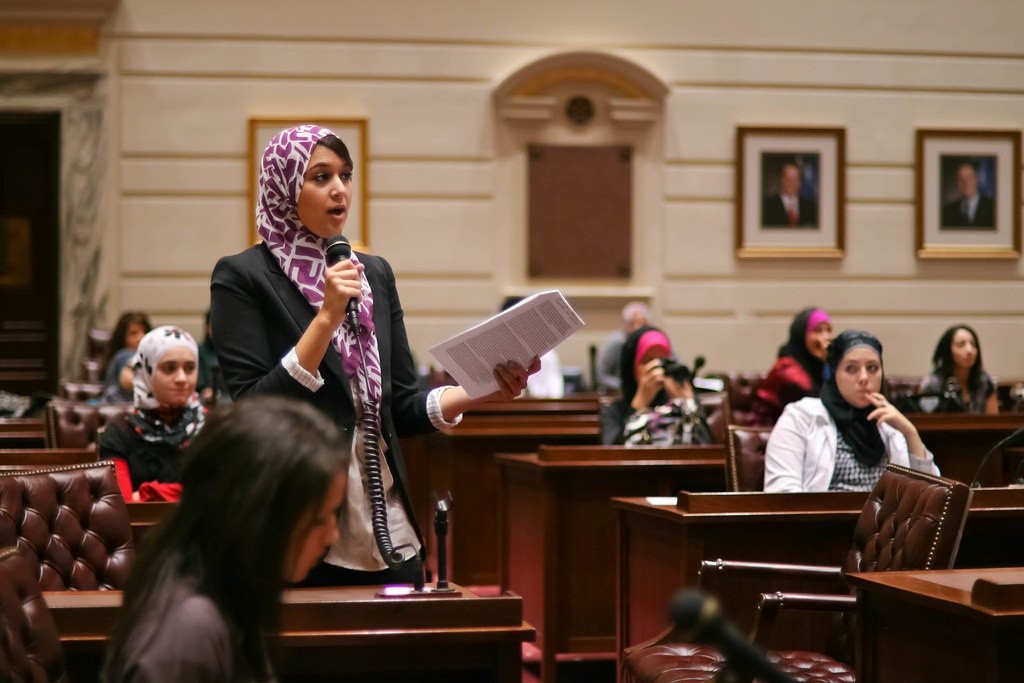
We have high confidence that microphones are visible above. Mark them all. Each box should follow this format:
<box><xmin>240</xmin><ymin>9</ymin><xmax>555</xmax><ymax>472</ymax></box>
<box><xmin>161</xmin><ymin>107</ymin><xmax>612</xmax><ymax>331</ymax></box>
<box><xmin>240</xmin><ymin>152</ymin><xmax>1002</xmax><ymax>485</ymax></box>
<box><xmin>326</xmin><ymin>235</ymin><xmax>360</xmax><ymax>337</ymax></box>
<box><xmin>669</xmin><ymin>589</ymin><xmax>800</xmax><ymax>683</ymax></box>
<box><xmin>689</xmin><ymin>356</ymin><xmax>716</xmax><ymax>443</ymax></box>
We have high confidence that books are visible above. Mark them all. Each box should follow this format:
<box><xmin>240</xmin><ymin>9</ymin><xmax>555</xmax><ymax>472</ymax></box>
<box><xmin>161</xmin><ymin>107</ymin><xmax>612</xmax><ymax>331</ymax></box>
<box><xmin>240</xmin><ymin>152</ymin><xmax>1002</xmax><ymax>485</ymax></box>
<box><xmin>429</xmin><ymin>291</ymin><xmax>586</xmax><ymax>399</ymax></box>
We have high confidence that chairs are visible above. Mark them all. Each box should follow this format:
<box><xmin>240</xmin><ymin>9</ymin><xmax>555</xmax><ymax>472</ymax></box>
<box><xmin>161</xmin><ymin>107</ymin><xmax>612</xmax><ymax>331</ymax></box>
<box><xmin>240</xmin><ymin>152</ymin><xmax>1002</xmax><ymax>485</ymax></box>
<box><xmin>45</xmin><ymin>328</ymin><xmax>136</xmax><ymax>449</ymax></box>
<box><xmin>621</xmin><ymin>463</ymin><xmax>974</xmax><ymax>683</ymax></box>
<box><xmin>724</xmin><ymin>421</ymin><xmax>773</xmax><ymax>491</ymax></box>
<box><xmin>881</xmin><ymin>376</ymin><xmax>923</xmax><ymax>413</ymax></box>
<box><xmin>1</xmin><ymin>549</ymin><xmax>77</xmax><ymax>683</ymax></box>
<box><xmin>722</xmin><ymin>372</ymin><xmax>774</xmax><ymax>427</ymax></box>
<box><xmin>0</xmin><ymin>461</ymin><xmax>136</xmax><ymax>592</ymax></box>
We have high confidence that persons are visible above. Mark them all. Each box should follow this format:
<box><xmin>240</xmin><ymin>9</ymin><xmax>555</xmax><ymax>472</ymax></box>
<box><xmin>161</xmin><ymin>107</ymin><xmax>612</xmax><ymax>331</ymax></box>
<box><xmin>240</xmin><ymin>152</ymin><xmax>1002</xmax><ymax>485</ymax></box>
<box><xmin>764</xmin><ymin>328</ymin><xmax>940</xmax><ymax>490</ymax></box>
<box><xmin>101</xmin><ymin>325</ymin><xmax>207</xmax><ymax>502</ymax></box>
<box><xmin>102</xmin><ymin>393</ymin><xmax>350</xmax><ymax>683</ymax></box>
<box><xmin>598</xmin><ymin>325</ymin><xmax>716</xmax><ymax>446</ymax></box>
<box><xmin>198</xmin><ymin>313</ymin><xmax>230</xmax><ymax>403</ymax></box>
<box><xmin>108</xmin><ymin>311</ymin><xmax>150</xmax><ymax>352</ymax></box>
<box><xmin>915</xmin><ymin>324</ymin><xmax>999</xmax><ymax>414</ymax></box>
<box><xmin>762</xmin><ymin>162</ymin><xmax>815</xmax><ymax>226</ymax></box>
<box><xmin>940</xmin><ymin>162</ymin><xmax>995</xmax><ymax>229</ymax></box>
<box><xmin>99</xmin><ymin>347</ymin><xmax>135</xmax><ymax>402</ymax></box>
<box><xmin>210</xmin><ymin>128</ymin><xmax>542</xmax><ymax>585</ymax></box>
<box><xmin>596</xmin><ymin>299</ymin><xmax>678</xmax><ymax>396</ymax></box>
<box><xmin>752</xmin><ymin>306</ymin><xmax>834</xmax><ymax>426</ymax></box>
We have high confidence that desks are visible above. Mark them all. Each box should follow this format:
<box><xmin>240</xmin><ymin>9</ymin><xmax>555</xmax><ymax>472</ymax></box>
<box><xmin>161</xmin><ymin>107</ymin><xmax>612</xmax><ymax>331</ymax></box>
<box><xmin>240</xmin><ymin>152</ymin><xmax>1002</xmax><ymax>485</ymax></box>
<box><xmin>0</xmin><ymin>380</ymin><xmax>1024</xmax><ymax>683</ymax></box>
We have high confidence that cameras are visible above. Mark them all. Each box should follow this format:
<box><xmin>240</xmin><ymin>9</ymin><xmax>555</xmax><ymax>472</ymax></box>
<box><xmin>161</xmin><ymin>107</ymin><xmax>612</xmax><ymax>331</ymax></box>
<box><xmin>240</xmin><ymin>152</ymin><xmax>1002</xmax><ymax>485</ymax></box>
<box><xmin>654</xmin><ymin>358</ymin><xmax>689</xmax><ymax>383</ymax></box>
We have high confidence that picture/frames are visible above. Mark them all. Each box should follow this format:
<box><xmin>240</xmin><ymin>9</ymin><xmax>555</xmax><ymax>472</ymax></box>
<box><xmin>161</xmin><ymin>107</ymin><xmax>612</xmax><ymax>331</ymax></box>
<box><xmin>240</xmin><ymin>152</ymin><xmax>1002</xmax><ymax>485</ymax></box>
<box><xmin>736</xmin><ymin>125</ymin><xmax>847</xmax><ymax>259</ymax></box>
<box><xmin>247</xmin><ymin>116</ymin><xmax>371</xmax><ymax>250</ymax></box>
<box><xmin>917</xmin><ymin>127</ymin><xmax>1024</xmax><ymax>259</ymax></box>
